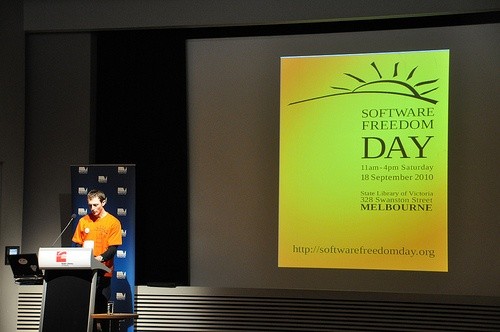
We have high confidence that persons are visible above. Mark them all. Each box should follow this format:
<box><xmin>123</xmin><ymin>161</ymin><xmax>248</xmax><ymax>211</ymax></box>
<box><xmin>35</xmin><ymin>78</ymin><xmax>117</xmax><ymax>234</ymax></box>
<box><xmin>71</xmin><ymin>188</ymin><xmax>123</xmax><ymax>332</ymax></box>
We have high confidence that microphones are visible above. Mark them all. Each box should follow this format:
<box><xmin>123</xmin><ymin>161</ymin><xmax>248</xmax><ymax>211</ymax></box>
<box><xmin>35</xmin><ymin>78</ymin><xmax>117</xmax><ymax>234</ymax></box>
<box><xmin>51</xmin><ymin>214</ymin><xmax>76</xmax><ymax>247</ymax></box>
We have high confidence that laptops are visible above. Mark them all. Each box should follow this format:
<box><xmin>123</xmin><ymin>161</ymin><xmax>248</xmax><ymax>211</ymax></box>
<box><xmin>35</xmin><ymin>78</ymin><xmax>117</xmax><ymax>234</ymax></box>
<box><xmin>8</xmin><ymin>254</ymin><xmax>42</xmax><ymax>277</ymax></box>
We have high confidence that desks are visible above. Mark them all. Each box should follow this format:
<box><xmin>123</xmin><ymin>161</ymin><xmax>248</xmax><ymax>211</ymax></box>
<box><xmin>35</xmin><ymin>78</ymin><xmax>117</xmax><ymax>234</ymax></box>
<box><xmin>90</xmin><ymin>313</ymin><xmax>138</xmax><ymax>332</ymax></box>
